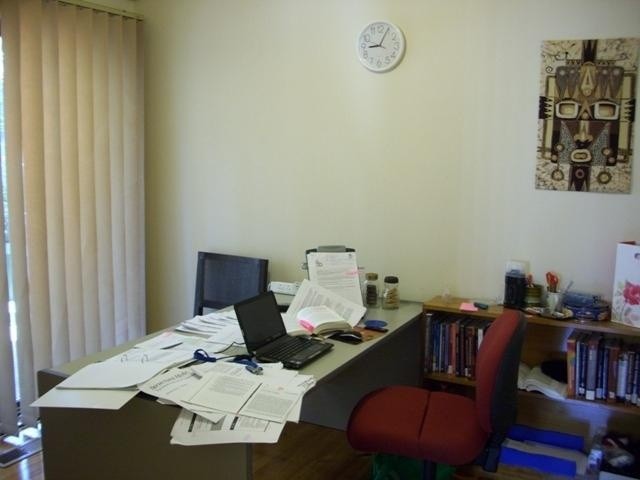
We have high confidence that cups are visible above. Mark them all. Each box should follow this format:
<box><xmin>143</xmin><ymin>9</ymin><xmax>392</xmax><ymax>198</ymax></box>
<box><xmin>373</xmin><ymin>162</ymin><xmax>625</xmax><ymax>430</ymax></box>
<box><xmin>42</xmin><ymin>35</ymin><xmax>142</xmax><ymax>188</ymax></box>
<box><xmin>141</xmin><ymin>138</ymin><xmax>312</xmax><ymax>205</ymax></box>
<box><xmin>547</xmin><ymin>291</ymin><xmax>562</xmax><ymax>313</ymax></box>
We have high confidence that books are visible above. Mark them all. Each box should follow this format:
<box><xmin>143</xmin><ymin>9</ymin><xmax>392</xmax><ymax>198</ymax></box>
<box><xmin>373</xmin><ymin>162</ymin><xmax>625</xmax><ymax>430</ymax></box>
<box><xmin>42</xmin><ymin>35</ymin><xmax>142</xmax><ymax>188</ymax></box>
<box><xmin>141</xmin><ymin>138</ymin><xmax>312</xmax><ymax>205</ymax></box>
<box><xmin>425</xmin><ymin>312</ymin><xmax>488</xmax><ymax>380</ymax></box>
<box><xmin>281</xmin><ymin>304</ymin><xmax>353</xmax><ymax>337</ymax></box>
<box><xmin>567</xmin><ymin>330</ymin><xmax>640</xmax><ymax>405</ymax></box>
<box><xmin>517</xmin><ymin>361</ymin><xmax>566</xmax><ymax>402</ymax></box>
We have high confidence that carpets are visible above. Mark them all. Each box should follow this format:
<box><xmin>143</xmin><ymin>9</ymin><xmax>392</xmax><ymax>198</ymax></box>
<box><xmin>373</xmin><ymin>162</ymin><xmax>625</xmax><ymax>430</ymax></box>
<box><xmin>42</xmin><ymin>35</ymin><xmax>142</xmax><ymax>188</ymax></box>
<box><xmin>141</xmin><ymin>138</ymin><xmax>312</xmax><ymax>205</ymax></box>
<box><xmin>0</xmin><ymin>420</ymin><xmax>42</xmax><ymax>469</ymax></box>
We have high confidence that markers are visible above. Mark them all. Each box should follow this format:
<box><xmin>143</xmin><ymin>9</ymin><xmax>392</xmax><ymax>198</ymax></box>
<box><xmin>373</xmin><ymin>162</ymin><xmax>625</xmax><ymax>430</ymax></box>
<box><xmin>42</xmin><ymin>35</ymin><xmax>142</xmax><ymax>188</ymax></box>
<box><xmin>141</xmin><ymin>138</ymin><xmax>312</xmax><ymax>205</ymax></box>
<box><xmin>474</xmin><ymin>303</ymin><xmax>488</xmax><ymax>310</ymax></box>
<box><xmin>299</xmin><ymin>319</ymin><xmax>316</xmax><ymax>332</ymax></box>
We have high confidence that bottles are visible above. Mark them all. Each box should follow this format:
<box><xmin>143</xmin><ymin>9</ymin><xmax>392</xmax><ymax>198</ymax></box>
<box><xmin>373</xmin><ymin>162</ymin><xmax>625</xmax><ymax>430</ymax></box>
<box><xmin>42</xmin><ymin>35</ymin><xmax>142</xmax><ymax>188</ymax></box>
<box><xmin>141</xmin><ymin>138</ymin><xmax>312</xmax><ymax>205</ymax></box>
<box><xmin>363</xmin><ymin>273</ymin><xmax>380</xmax><ymax>308</ymax></box>
<box><xmin>381</xmin><ymin>275</ymin><xmax>400</xmax><ymax>309</ymax></box>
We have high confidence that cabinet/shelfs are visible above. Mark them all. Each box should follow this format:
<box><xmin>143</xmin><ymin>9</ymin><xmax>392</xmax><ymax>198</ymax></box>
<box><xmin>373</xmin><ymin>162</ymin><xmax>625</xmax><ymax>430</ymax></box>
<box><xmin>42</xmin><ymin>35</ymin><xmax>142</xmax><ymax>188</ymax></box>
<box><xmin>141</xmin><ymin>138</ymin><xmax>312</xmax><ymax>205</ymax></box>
<box><xmin>422</xmin><ymin>294</ymin><xmax>639</xmax><ymax>480</ymax></box>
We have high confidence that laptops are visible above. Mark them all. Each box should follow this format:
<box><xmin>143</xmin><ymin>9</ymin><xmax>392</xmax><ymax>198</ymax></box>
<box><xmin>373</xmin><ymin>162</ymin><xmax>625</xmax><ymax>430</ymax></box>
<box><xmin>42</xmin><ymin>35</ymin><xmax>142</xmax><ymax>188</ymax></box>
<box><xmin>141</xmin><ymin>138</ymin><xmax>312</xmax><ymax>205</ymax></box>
<box><xmin>232</xmin><ymin>290</ymin><xmax>334</xmax><ymax>370</ymax></box>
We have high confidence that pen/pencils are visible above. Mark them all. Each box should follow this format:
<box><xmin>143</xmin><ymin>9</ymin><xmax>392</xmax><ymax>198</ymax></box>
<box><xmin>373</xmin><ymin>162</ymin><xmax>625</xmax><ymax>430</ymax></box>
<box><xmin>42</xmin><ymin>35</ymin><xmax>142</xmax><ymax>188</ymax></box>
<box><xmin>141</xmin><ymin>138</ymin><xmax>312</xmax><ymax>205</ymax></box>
<box><xmin>561</xmin><ymin>280</ymin><xmax>573</xmax><ymax>294</ymax></box>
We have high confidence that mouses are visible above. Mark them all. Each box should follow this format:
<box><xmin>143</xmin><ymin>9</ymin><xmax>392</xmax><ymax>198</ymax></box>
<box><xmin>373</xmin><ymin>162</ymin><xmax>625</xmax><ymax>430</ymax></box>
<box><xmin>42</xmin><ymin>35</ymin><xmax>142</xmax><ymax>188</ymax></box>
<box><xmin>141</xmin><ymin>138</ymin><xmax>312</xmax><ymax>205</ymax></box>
<box><xmin>327</xmin><ymin>331</ymin><xmax>362</xmax><ymax>344</ymax></box>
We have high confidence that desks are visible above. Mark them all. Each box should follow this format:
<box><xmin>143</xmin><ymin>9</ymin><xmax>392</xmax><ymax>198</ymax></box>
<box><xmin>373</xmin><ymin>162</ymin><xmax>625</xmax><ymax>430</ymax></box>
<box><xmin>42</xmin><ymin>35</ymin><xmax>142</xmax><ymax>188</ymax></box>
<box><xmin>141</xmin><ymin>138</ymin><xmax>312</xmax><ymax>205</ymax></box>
<box><xmin>37</xmin><ymin>291</ymin><xmax>424</xmax><ymax>480</ymax></box>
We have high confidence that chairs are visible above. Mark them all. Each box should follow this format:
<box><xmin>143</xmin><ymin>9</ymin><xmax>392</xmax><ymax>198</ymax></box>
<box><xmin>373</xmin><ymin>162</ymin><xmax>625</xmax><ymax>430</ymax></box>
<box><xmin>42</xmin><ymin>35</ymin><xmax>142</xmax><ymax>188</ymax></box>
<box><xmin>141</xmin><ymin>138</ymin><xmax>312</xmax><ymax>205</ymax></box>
<box><xmin>193</xmin><ymin>251</ymin><xmax>269</xmax><ymax>317</ymax></box>
<box><xmin>346</xmin><ymin>309</ymin><xmax>526</xmax><ymax>479</ymax></box>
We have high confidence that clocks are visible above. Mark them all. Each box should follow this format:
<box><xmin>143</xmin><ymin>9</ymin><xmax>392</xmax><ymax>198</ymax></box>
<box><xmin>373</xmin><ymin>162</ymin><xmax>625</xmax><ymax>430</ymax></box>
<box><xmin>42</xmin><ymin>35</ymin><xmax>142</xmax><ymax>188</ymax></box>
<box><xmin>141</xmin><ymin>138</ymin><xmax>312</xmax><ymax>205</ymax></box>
<box><xmin>355</xmin><ymin>20</ymin><xmax>407</xmax><ymax>73</ymax></box>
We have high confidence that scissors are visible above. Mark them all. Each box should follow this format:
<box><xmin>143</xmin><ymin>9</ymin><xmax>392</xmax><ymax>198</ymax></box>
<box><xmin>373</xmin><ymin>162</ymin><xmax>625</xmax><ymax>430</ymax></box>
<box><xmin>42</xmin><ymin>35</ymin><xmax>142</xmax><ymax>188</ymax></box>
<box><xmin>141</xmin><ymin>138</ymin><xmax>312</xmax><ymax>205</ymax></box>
<box><xmin>546</xmin><ymin>272</ymin><xmax>558</xmax><ymax>293</ymax></box>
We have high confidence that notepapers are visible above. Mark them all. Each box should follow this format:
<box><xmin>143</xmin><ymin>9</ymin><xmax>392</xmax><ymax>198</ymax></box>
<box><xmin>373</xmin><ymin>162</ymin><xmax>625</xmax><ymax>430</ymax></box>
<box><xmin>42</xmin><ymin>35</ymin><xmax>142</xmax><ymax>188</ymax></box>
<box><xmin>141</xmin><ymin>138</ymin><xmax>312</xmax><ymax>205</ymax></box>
<box><xmin>459</xmin><ymin>302</ymin><xmax>478</xmax><ymax>312</ymax></box>
